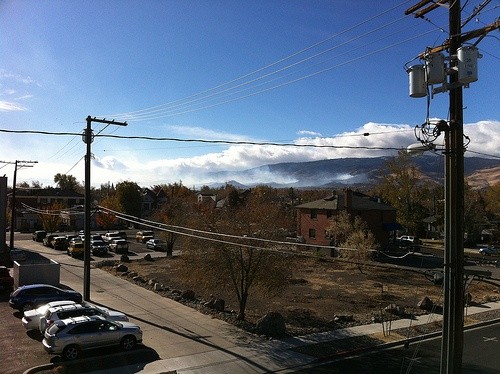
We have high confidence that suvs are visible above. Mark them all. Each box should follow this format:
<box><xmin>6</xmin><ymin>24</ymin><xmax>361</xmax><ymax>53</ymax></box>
<box><xmin>8</xmin><ymin>284</ymin><xmax>83</xmax><ymax>314</ymax></box>
<box><xmin>479</xmin><ymin>245</ymin><xmax>500</xmax><ymax>256</ymax></box>
<box><xmin>41</xmin><ymin>315</ymin><xmax>143</xmax><ymax>361</ymax></box>
<box><xmin>33</xmin><ymin>230</ymin><xmax>129</xmax><ymax>259</ymax></box>
<box><xmin>397</xmin><ymin>235</ymin><xmax>419</xmax><ymax>243</ymax></box>
<box><xmin>39</xmin><ymin>302</ymin><xmax>129</xmax><ymax>335</ymax></box>
<box><xmin>135</xmin><ymin>231</ymin><xmax>155</xmax><ymax>243</ymax></box>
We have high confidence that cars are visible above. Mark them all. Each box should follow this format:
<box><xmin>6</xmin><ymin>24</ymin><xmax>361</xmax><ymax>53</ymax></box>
<box><xmin>145</xmin><ymin>239</ymin><xmax>167</xmax><ymax>251</ymax></box>
<box><xmin>21</xmin><ymin>300</ymin><xmax>75</xmax><ymax>331</ymax></box>
